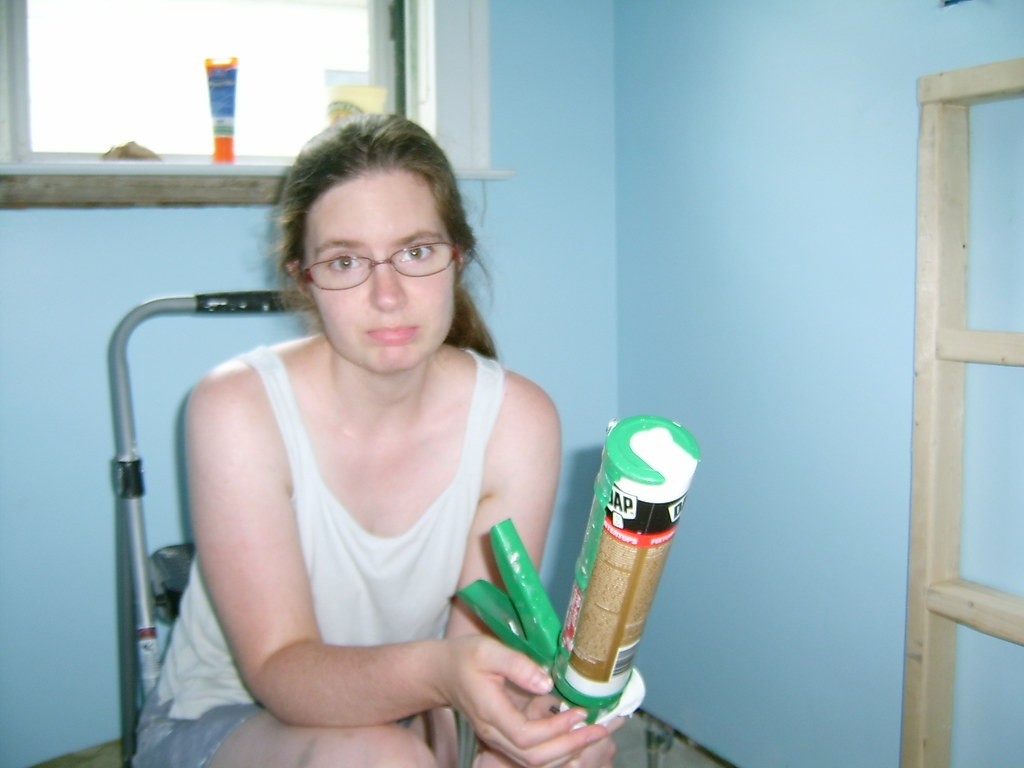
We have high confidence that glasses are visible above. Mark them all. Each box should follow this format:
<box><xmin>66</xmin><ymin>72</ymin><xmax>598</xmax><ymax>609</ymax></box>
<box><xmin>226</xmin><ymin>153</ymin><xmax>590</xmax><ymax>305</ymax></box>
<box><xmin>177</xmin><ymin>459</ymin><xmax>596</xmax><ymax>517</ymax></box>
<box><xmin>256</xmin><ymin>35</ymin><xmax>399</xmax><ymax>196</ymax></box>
<box><xmin>297</xmin><ymin>240</ymin><xmax>462</xmax><ymax>291</ymax></box>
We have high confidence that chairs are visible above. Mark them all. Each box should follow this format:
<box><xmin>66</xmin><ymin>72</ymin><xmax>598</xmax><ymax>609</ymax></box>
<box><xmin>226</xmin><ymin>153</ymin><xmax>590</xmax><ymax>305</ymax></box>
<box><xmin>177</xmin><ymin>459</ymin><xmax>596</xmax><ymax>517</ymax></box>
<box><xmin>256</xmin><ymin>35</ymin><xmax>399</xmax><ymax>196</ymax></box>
<box><xmin>109</xmin><ymin>290</ymin><xmax>313</xmax><ymax>768</ymax></box>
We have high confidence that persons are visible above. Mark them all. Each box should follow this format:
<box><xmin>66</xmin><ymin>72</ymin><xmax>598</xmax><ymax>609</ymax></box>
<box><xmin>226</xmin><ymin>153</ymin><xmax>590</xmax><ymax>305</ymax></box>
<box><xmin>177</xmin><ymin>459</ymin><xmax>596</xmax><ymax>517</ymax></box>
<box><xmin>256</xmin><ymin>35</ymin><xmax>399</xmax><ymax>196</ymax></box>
<box><xmin>133</xmin><ymin>111</ymin><xmax>623</xmax><ymax>768</ymax></box>
